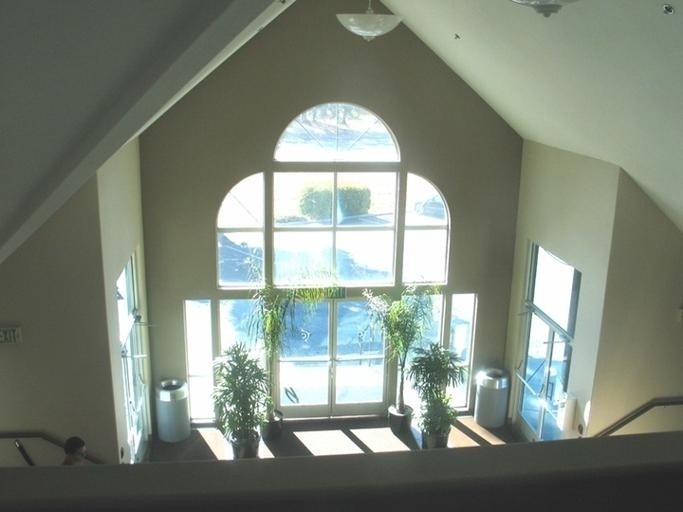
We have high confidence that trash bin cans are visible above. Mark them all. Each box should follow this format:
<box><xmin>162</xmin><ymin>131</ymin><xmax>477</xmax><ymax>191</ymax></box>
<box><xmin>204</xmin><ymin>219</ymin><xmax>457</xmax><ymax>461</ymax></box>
<box><xmin>154</xmin><ymin>379</ymin><xmax>190</xmax><ymax>443</ymax></box>
<box><xmin>475</xmin><ymin>368</ymin><xmax>510</xmax><ymax>429</ymax></box>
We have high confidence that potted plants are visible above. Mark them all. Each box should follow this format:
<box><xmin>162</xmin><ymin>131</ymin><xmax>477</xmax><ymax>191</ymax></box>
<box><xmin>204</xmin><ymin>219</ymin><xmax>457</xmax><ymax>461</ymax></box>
<box><xmin>209</xmin><ymin>242</ymin><xmax>346</xmax><ymax>462</ymax></box>
<box><xmin>359</xmin><ymin>278</ymin><xmax>469</xmax><ymax>449</ymax></box>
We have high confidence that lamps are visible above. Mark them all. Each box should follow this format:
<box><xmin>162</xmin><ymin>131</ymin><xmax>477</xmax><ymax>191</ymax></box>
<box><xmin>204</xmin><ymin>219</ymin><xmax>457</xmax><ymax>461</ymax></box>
<box><xmin>334</xmin><ymin>1</ymin><xmax>402</xmax><ymax>43</ymax></box>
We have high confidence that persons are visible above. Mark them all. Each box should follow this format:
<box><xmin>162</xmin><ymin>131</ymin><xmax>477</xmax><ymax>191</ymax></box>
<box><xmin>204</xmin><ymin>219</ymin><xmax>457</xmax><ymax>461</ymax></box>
<box><xmin>61</xmin><ymin>436</ymin><xmax>86</xmax><ymax>465</ymax></box>
